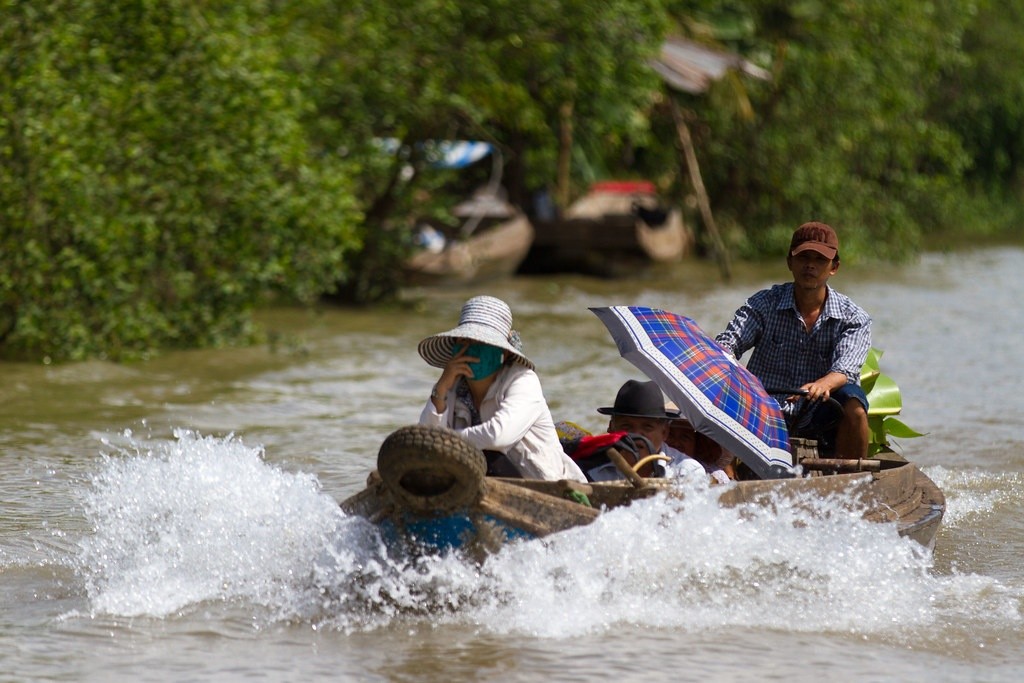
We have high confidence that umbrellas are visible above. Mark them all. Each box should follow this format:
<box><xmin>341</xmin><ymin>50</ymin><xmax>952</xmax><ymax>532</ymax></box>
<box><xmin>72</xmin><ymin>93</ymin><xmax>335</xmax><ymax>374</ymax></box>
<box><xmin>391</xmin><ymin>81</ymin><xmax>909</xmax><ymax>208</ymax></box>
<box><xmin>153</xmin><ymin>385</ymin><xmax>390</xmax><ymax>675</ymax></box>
<box><xmin>588</xmin><ymin>307</ymin><xmax>793</xmax><ymax>480</ymax></box>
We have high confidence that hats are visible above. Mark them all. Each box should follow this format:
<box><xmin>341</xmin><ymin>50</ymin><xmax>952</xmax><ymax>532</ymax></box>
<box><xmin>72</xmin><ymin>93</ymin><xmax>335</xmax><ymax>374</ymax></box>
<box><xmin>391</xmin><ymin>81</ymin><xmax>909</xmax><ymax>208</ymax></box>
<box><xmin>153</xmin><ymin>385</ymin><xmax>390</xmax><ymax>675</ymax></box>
<box><xmin>664</xmin><ymin>402</ymin><xmax>734</xmax><ymax>473</ymax></box>
<box><xmin>791</xmin><ymin>222</ymin><xmax>839</xmax><ymax>260</ymax></box>
<box><xmin>418</xmin><ymin>295</ymin><xmax>536</xmax><ymax>372</ymax></box>
<box><xmin>597</xmin><ymin>380</ymin><xmax>682</xmax><ymax>420</ymax></box>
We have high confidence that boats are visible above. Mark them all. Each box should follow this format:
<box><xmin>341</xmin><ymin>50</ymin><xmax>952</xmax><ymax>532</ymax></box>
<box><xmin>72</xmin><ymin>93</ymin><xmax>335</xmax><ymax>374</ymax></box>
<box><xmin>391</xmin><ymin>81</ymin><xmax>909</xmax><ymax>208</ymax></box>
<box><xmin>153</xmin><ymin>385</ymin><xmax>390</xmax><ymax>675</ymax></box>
<box><xmin>543</xmin><ymin>181</ymin><xmax>697</xmax><ymax>277</ymax></box>
<box><xmin>405</xmin><ymin>205</ymin><xmax>533</xmax><ymax>288</ymax></box>
<box><xmin>334</xmin><ymin>425</ymin><xmax>946</xmax><ymax>567</ymax></box>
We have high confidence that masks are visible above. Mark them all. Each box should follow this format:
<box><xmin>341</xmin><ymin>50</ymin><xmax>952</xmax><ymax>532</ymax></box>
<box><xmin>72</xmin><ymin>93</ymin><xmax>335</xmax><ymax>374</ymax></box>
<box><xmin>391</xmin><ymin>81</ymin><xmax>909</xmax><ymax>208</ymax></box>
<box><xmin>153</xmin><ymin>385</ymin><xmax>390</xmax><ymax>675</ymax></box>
<box><xmin>452</xmin><ymin>344</ymin><xmax>505</xmax><ymax>380</ymax></box>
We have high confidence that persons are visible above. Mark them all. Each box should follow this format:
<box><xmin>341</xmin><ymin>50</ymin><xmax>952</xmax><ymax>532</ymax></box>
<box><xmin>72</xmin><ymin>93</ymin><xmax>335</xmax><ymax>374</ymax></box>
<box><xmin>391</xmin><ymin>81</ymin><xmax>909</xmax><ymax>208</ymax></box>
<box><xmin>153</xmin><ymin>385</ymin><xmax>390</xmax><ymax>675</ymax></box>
<box><xmin>716</xmin><ymin>222</ymin><xmax>873</xmax><ymax>474</ymax></box>
<box><xmin>596</xmin><ymin>379</ymin><xmax>706</xmax><ymax>487</ymax></box>
<box><xmin>417</xmin><ymin>295</ymin><xmax>587</xmax><ymax>485</ymax></box>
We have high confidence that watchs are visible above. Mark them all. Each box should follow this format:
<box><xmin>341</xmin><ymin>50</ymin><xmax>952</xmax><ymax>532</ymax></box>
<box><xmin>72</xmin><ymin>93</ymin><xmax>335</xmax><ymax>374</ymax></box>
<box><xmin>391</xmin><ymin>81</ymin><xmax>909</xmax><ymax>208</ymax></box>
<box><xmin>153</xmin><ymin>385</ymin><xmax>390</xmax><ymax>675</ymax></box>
<box><xmin>432</xmin><ymin>387</ymin><xmax>448</xmax><ymax>400</ymax></box>
